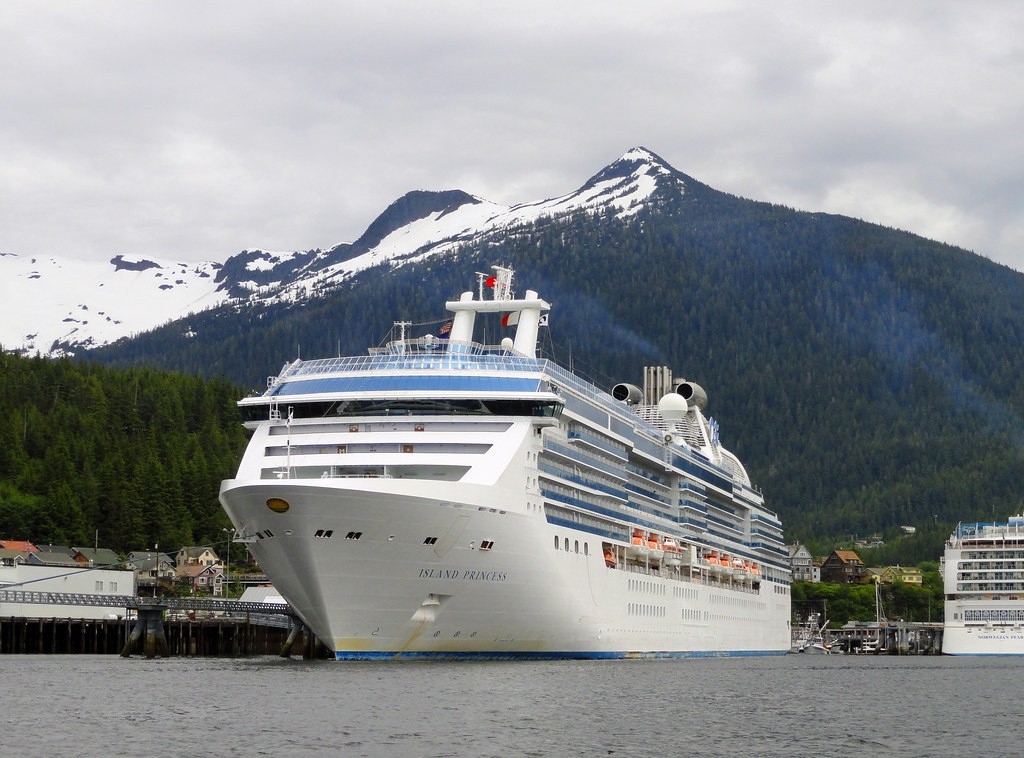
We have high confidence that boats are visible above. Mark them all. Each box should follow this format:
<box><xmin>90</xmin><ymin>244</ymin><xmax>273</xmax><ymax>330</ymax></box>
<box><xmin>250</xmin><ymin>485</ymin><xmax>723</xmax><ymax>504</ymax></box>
<box><xmin>798</xmin><ymin>609</ymin><xmax>829</xmax><ymax>655</ymax></box>
<box><xmin>219</xmin><ymin>264</ymin><xmax>796</xmax><ymax>660</ymax></box>
